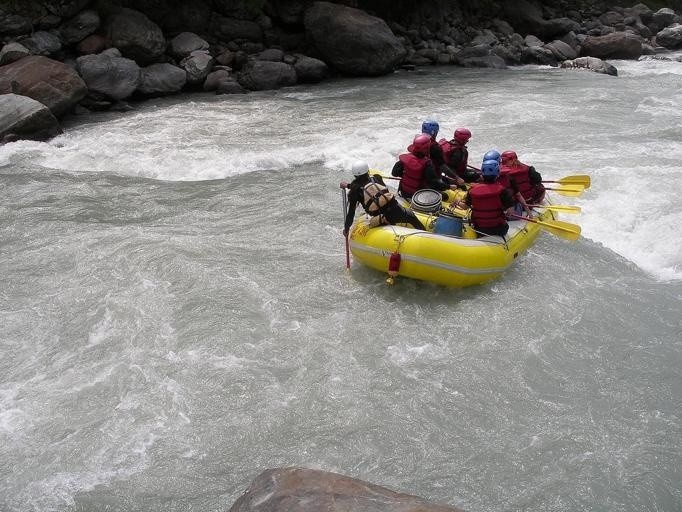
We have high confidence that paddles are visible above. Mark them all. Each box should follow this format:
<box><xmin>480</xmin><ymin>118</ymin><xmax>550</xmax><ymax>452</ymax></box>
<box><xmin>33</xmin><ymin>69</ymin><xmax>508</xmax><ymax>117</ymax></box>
<box><xmin>511</xmin><ymin>213</ymin><xmax>581</xmax><ymax>241</ymax></box>
<box><xmin>544</xmin><ymin>185</ymin><xmax>584</xmax><ymax>197</ymax></box>
<box><xmin>541</xmin><ymin>175</ymin><xmax>590</xmax><ymax>189</ymax></box>
<box><xmin>368</xmin><ymin>168</ymin><xmax>402</xmax><ymax>180</ymax></box>
<box><xmin>528</xmin><ymin>204</ymin><xmax>581</xmax><ymax>215</ymax></box>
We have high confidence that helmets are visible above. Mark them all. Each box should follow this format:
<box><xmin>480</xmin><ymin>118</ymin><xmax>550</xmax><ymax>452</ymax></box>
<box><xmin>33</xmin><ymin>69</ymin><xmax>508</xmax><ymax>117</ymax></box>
<box><xmin>481</xmin><ymin>150</ymin><xmax>517</xmax><ymax>176</ymax></box>
<box><xmin>415</xmin><ymin>136</ymin><xmax>430</xmax><ymax>151</ymax></box>
<box><xmin>422</xmin><ymin>121</ymin><xmax>439</xmax><ymax>135</ymax></box>
<box><xmin>352</xmin><ymin>161</ymin><xmax>369</xmax><ymax>177</ymax></box>
<box><xmin>455</xmin><ymin>128</ymin><xmax>471</xmax><ymax>144</ymax></box>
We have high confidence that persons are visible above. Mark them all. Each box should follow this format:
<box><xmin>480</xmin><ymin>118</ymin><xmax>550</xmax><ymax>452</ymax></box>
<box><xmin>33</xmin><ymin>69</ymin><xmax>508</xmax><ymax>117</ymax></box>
<box><xmin>392</xmin><ymin>135</ymin><xmax>448</xmax><ymax>202</ymax></box>
<box><xmin>340</xmin><ymin>160</ymin><xmax>427</xmax><ymax>237</ymax></box>
<box><xmin>483</xmin><ymin>149</ymin><xmax>534</xmax><ymax>221</ymax></box>
<box><xmin>501</xmin><ymin>150</ymin><xmax>545</xmax><ymax>204</ymax></box>
<box><xmin>438</xmin><ymin>127</ymin><xmax>479</xmax><ymax>182</ymax></box>
<box><xmin>422</xmin><ymin>118</ymin><xmax>465</xmax><ymax>186</ymax></box>
<box><xmin>454</xmin><ymin>159</ymin><xmax>513</xmax><ymax>237</ymax></box>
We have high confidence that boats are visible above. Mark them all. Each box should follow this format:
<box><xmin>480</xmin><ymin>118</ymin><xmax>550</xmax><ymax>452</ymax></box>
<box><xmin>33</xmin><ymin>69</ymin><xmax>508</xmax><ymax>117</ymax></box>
<box><xmin>346</xmin><ymin>189</ymin><xmax>561</xmax><ymax>287</ymax></box>
